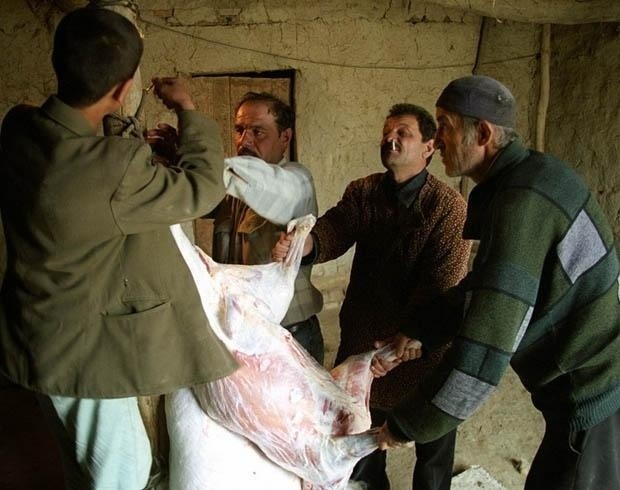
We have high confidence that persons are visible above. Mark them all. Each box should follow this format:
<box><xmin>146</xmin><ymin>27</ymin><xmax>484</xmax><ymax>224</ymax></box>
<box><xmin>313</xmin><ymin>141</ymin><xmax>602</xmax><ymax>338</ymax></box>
<box><xmin>146</xmin><ymin>91</ymin><xmax>323</xmax><ymax>367</ymax></box>
<box><xmin>378</xmin><ymin>76</ymin><xmax>620</xmax><ymax>490</ymax></box>
<box><xmin>272</xmin><ymin>103</ymin><xmax>471</xmax><ymax>490</ymax></box>
<box><xmin>0</xmin><ymin>8</ymin><xmax>240</xmax><ymax>490</ymax></box>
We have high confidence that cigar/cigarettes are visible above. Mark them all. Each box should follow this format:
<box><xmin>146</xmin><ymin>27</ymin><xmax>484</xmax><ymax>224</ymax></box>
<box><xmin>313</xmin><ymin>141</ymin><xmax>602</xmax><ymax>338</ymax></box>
<box><xmin>391</xmin><ymin>140</ymin><xmax>395</xmax><ymax>151</ymax></box>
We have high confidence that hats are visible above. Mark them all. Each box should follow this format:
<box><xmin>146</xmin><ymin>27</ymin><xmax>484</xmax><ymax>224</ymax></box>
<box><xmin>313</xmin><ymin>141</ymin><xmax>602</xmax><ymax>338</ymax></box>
<box><xmin>436</xmin><ymin>75</ymin><xmax>517</xmax><ymax>128</ymax></box>
